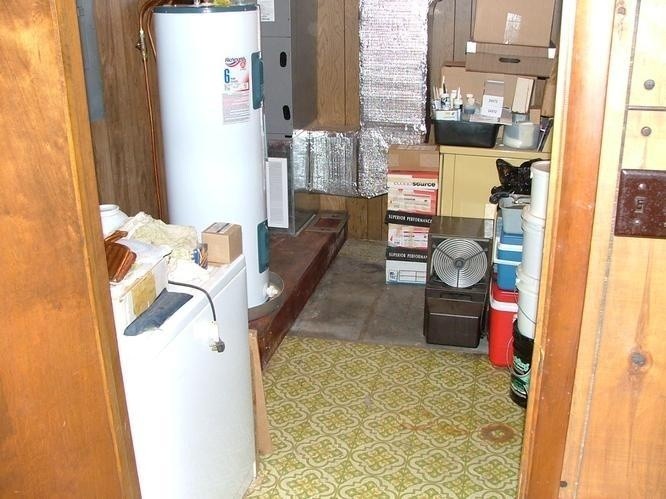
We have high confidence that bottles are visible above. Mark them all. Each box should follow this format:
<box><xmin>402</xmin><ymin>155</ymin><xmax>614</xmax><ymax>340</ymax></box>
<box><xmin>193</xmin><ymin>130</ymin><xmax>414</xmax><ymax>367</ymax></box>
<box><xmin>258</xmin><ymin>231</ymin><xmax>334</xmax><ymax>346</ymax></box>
<box><xmin>435</xmin><ymin>88</ymin><xmax>476</xmax><ymax>114</ymax></box>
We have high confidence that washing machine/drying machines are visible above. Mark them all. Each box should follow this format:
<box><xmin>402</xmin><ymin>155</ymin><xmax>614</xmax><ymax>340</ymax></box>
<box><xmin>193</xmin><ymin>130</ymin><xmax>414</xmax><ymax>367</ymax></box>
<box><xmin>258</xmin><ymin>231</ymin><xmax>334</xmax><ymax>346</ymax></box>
<box><xmin>106</xmin><ymin>254</ymin><xmax>259</xmax><ymax>499</ymax></box>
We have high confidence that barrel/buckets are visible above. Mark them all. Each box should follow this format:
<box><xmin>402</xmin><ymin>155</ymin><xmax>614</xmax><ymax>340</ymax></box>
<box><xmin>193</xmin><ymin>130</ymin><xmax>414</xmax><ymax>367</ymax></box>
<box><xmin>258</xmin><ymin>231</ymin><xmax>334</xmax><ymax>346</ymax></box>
<box><xmin>514</xmin><ymin>269</ymin><xmax>541</xmax><ymax>341</ymax></box>
<box><xmin>529</xmin><ymin>160</ymin><xmax>551</xmax><ymax>221</ymax></box>
<box><xmin>505</xmin><ymin>318</ymin><xmax>533</xmax><ymax>411</ymax></box>
<box><xmin>518</xmin><ymin>203</ymin><xmax>547</xmax><ymax>280</ymax></box>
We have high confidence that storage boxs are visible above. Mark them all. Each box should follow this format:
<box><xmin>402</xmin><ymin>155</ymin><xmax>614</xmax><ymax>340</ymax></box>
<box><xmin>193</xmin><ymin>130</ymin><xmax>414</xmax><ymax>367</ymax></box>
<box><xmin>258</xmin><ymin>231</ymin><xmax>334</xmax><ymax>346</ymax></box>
<box><xmin>499</xmin><ymin>198</ymin><xmax>526</xmax><ymax>234</ymax></box>
<box><xmin>388</xmin><ymin>186</ymin><xmax>437</xmax><ymax>215</ymax></box>
<box><xmin>433</xmin><ymin>118</ymin><xmax>502</xmax><ymax>148</ymax></box>
<box><xmin>493</xmin><ymin>229</ymin><xmax>524</xmax><ymax>293</ymax></box>
<box><xmin>464</xmin><ymin>40</ymin><xmax>556</xmax><ymax>79</ymax></box>
<box><xmin>385</xmin><ymin>248</ymin><xmax>427</xmax><ymax>285</ymax></box>
<box><xmin>388</xmin><ymin>144</ymin><xmax>440</xmax><ymax>171</ymax></box>
<box><xmin>440</xmin><ymin>60</ymin><xmax>538</xmax><ymax>112</ymax></box>
<box><xmin>471</xmin><ymin>1</ymin><xmax>556</xmax><ymax>47</ymax></box>
<box><xmin>488</xmin><ymin>276</ymin><xmax>520</xmax><ymax>368</ymax></box>
<box><xmin>386</xmin><ymin>172</ymin><xmax>439</xmax><ymax>190</ymax></box>
<box><xmin>384</xmin><ymin>211</ymin><xmax>430</xmax><ymax>247</ymax></box>
<box><xmin>108</xmin><ymin>240</ymin><xmax>169</xmax><ymax>334</ymax></box>
